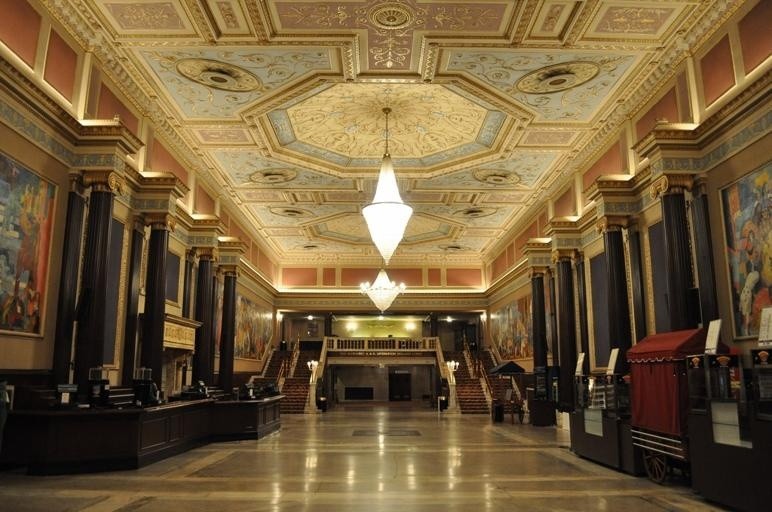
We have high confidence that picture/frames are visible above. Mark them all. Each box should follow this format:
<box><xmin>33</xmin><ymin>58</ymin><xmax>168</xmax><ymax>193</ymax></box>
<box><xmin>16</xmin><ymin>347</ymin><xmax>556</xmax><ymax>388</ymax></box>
<box><xmin>717</xmin><ymin>159</ymin><xmax>771</xmax><ymax>342</ymax></box>
<box><xmin>0</xmin><ymin>148</ymin><xmax>60</xmax><ymax>341</ymax></box>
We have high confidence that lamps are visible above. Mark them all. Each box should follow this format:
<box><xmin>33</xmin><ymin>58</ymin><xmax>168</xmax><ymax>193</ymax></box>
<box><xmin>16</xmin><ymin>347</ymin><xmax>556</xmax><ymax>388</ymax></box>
<box><xmin>360</xmin><ymin>107</ymin><xmax>413</xmax><ymax>265</ymax></box>
<box><xmin>358</xmin><ymin>257</ymin><xmax>406</xmax><ymax>315</ymax></box>
